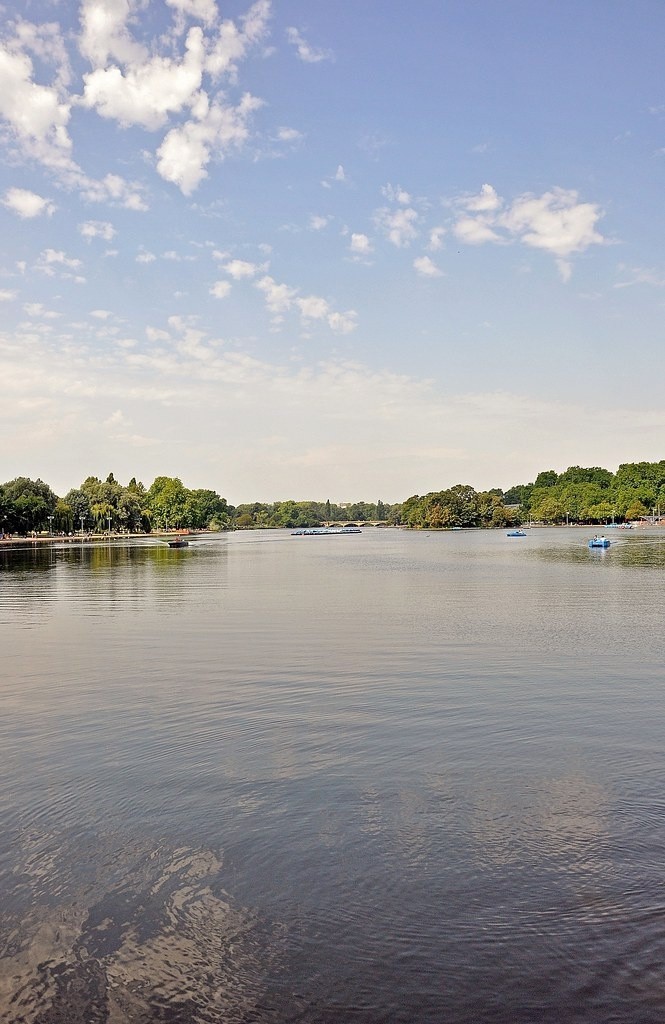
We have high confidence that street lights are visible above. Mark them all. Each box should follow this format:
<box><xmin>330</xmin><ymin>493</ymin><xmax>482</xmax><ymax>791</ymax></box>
<box><xmin>46</xmin><ymin>516</ymin><xmax>55</xmax><ymax>537</ymax></box>
<box><xmin>106</xmin><ymin>517</ymin><xmax>113</xmax><ymax>536</ymax></box>
<box><xmin>79</xmin><ymin>516</ymin><xmax>86</xmax><ymax>538</ymax></box>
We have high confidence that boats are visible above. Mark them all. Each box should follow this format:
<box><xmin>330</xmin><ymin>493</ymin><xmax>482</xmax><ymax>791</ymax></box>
<box><xmin>588</xmin><ymin>539</ymin><xmax>610</xmax><ymax>548</ymax></box>
<box><xmin>606</xmin><ymin>523</ymin><xmax>632</xmax><ymax>529</ymax></box>
<box><xmin>167</xmin><ymin>539</ymin><xmax>188</xmax><ymax>549</ymax></box>
<box><xmin>290</xmin><ymin>527</ymin><xmax>363</xmax><ymax>536</ymax></box>
<box><xmin>506</xmin><ymin>531</ymin><xmax>527</xmax><ymax>536</ymax></box>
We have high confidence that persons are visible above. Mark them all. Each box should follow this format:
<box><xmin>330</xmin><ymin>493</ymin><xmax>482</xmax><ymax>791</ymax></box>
<box><xmin>518</xmin><ymin>529</ymin><xmax>607</xmax><ymax>542</ymax></box>
